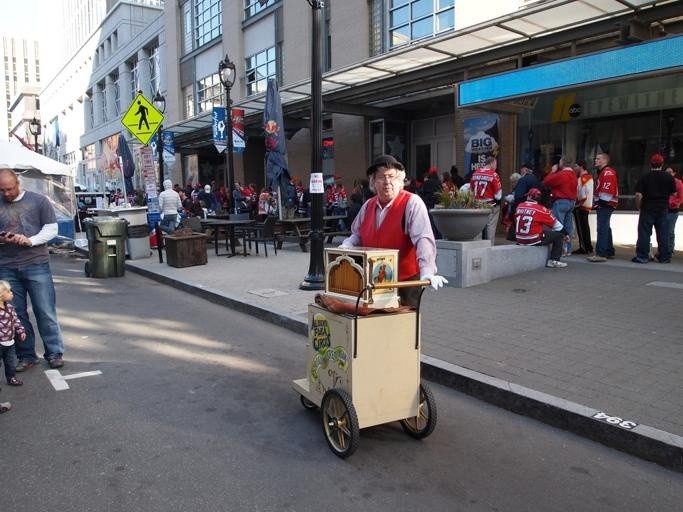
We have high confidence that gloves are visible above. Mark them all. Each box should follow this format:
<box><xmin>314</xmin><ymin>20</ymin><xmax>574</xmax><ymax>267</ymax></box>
<box><xmin>337</xmin><ymin>242</ymin><xmax>353</xmax><ymax>249</ymax></box>
<box><xmin>419</xmin><ymin>274</ymin><xmax>449</xmax><ymax>291</ymax></box>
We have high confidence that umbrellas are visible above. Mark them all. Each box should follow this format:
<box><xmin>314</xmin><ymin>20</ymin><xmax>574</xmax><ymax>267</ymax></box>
<box><xmin>0</xmin><ymin>134</ymin><xmax>71</xmax><ymax>176</ymax></box>
<box><xmin>263</xmin><ymin>78</ymin><xmax>290</xmax><ymax>221</ymax></box>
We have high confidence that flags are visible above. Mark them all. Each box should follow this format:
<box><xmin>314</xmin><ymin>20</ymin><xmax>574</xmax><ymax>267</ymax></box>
<box><xmin>160</xmin><ymin>131</ymin><xmax>175</xmax><ymax>169</ymax></box>
<box><xmin>212</xmin><ymin>106</ymin><xmax>228</xmax><ymax>153</ymax></box>
<box><xmin>231</xmin><ymin>108</ymin><xmax>247</xmax><ymax>156</ymax></box>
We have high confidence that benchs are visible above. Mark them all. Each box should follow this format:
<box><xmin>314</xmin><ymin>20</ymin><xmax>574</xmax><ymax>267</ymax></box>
<box><xmin>275</xmin><ymin>216</ymin><xmax>352</xmax><ymax>252</ymax></box>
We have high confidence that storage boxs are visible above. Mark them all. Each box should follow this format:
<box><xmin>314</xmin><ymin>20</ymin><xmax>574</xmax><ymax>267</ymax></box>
<box><xmin>324</xmin><ymin>248</ymin><xmax>400</xmax><ymax>308</ymax></box>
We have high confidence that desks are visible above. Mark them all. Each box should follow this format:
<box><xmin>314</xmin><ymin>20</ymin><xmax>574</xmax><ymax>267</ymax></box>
<box><xmin>207</xmin><ymin>220</ymin><xmax>257</xmax><ymax>258</ymax></box>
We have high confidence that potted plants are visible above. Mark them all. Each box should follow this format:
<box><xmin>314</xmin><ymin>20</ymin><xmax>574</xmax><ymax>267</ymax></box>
<box><xmin>429</xmin><ymin>181</ymin><xmax>493</xmax><ymax>241</ymax></box>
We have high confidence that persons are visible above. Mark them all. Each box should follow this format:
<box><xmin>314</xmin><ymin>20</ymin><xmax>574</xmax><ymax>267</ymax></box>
<box><xmin>450</xmin><ymin>166</ymin><xmax>466</xmax><ymax>201</ymax></box>
<box><xmin>586</xmin><ymin>153</ymin><xmax>619</xmax><ymax>262</ymax></box>
<box><xmin>572</xmin><ymin>158</ymin><xmax>594</xmax><ymax>255</ymax></box>
<box><xmin>505</xmin><ymin>162</ymin><xmax>539</xmax><ymax>243</ymax></box>
<box><xmin>283</xmin><ymin>178</ymin><xmax>370</xmax><ymax>218</ymax></box>
<box><xmin>631</xmin><ymin>153</ymin><xmax>677</xmax><ymax>264</ymax></box>
<box><xmin>663</xmin><ymin>164</ymin><xmax>683</xmax><ymax>256</ymax></box>
<box><xmin>159</xmin><ymin>178</ymin><xmax>183</xmax><ymax>236</ymax></box>
<box><xmin>175</xmin><ymin>183</ymin><xmax>279</xmax><ymax>219</ymax></box>
<box><xmin>418</xmin><ymin>165</ymin><xmax>443</xmax><ymax>239</ymax></box>
<box><xmin>116</xmin><ymin>188</ymin><xmax>124</xmax><ymax>204</ymax></box>
<box><xmin>405</xmin><ymin>177</ymin><xmax>411</xmax><ymax>190</ymax></box>
<box><xmin>542</xmin><ymin>155</ymin><xmax>578</xmax><ymax>257</ymax></box>
<box><xmin>0</xmin><ymin>168</ymin><xmax>64</xmax><ymax>372</ymax></box>
<box><xmin>413</xmin><ymin>178</ymin><xmax>423</xmax><ymax>191</ymax></box>
<box><xmin>338</xmin><ymin>155</ymin><xmax>448</xmax><ymax>311</ymax></box>
<box><xmin>0</xmin><ymin>280</ymin><xmax>26</xmax><ymax>386</ymax></box>
<box><xmin>440</xmin><ymin>171</ymin><xmax>458</xmax><ymax>202</ymax></box>
<box><xmin>111</xmin><ymin>191</ymin><xmax>114</xmax><ymax>202</ymax></box>
<box><xmin>507</xmin><ymin>169</ymin><xmax>520</xmax><ymax>192</ymax></box>
<box><xmin>513</xmin><ymin>188</ymin><xmax>568</xmax><ymax>268</ymax></box>
<box><xmin>469</xmin><ymin>156</ymin><xmax>503</xmax><ymax>246</ymax></box>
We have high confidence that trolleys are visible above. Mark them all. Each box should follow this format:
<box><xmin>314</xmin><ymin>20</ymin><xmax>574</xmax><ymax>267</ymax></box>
<box><xmin>291</xmin><ymin>279</ymin><xmax>439</xmax><ymax>460</ymax></box>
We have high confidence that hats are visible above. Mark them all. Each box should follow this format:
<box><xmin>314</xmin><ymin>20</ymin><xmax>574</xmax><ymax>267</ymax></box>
<box><xmin>205</xmin><ymin>185</ymin><xmax>211</xmax><ymax>194</ymax></box>
<box><xmin>649</xmin><ymin>154</ymin><xmax>663</xmax><ymax>165</ymax></box>
<box><xmin>524</xmin><ymin>188</ymin><xmax>542</xmax><ymax>198</ymax></box>
<box><xmin>366</xmin><ymin>154</ymin><xmax>405</xmax><ymax>176</ymax></box>
<box><xmin>429</xmin><ymin>165</ymin><xmax>438</xmax><ymax>175</ymax></box>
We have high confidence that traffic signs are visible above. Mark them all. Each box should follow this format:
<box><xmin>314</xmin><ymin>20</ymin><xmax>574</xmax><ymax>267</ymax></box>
<box><xmin>122</xmin><ymin>91</ymin><xmax>163</xmax><ymax>146</ymax></box>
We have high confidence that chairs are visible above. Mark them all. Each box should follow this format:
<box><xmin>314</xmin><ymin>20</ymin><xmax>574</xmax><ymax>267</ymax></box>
<box><xmin>242</xmin><ymin>215</ymin><xmax>277</xmax><ymax>257</ymax></box>
<box><xmin>230</xmin><ymin>213</ymin><xmax>251</xmax><ymax>250</ymax></box>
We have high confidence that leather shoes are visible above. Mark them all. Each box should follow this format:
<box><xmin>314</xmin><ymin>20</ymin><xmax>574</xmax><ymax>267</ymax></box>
<box><xmin>587</xmin><ymin>255</ymin><xmax>606</xmax><ymax>262</ymax></box>
<box><xmin>1</xmin><ymin>401</ymin><xmax>12</xmax><ymax>413</ymax></box>
<box><xmin>49</xmin><ymin>355</ymin><xmax>63</xmax><ymax>368</ymax></box>
<box><xmin>8</xmin><ymin>376</ymin><xmax>21</xmax><ymax>386</ymax></box>
<box><xmin>607</xmin><ymin>254</ymin><xmax>615</xmax><ymax>259</ymax></box>
<box><xmin>14</xmin><ymin>357</ymin><xmax>39</xmax><ymax>371</ymax></box>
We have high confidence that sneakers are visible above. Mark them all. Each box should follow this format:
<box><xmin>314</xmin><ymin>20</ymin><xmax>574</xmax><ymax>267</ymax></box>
<box><xmin>547</xmin><ymin>259</ymin><xmax>553</xmax><ymax>266</ymax></box>
<box><xmin>551</xmin><ymin>261</ymin><xmax>568</xmax><ymax>268</ymax></box>
<box><xmin>632</xmin><ymin>256</ymin><xmax>649</xmax><ymax>263</ymax></box>
<box><xmin>655</xmin><ymin>256</ymin><xmax>669</xmax><ymax>262</ymax></box>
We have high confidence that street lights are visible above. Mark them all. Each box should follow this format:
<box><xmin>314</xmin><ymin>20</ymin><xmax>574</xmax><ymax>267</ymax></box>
<box><xmin>151</xmin><ymin>90</ymin><xmax>166</xmax><ymax>192</ymax></box>
<box><xmin>29</xmin><ymin>117</ymin><xmax>40</xmax><ymax>152</ymax></box>
<box><xmin>218</xmin><ymin>55</ymin><xmax>236</xmax><ymax>212</ymax></box>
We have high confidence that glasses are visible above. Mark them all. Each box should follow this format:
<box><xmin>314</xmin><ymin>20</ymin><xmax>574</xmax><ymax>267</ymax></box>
<box><xmin>372</xmin><ymin>177</ymin><xmax>400</xmax><ymax>183</ymax></box>
<box><xmin>0</xmin><ymin>184</ymin><xmax>17</xmax><ymax>194</ymax></box>
<box><xmin>403</xmin><ymin>180</ymin><xmax>408</xmax><ymax>183</ymax></box>
